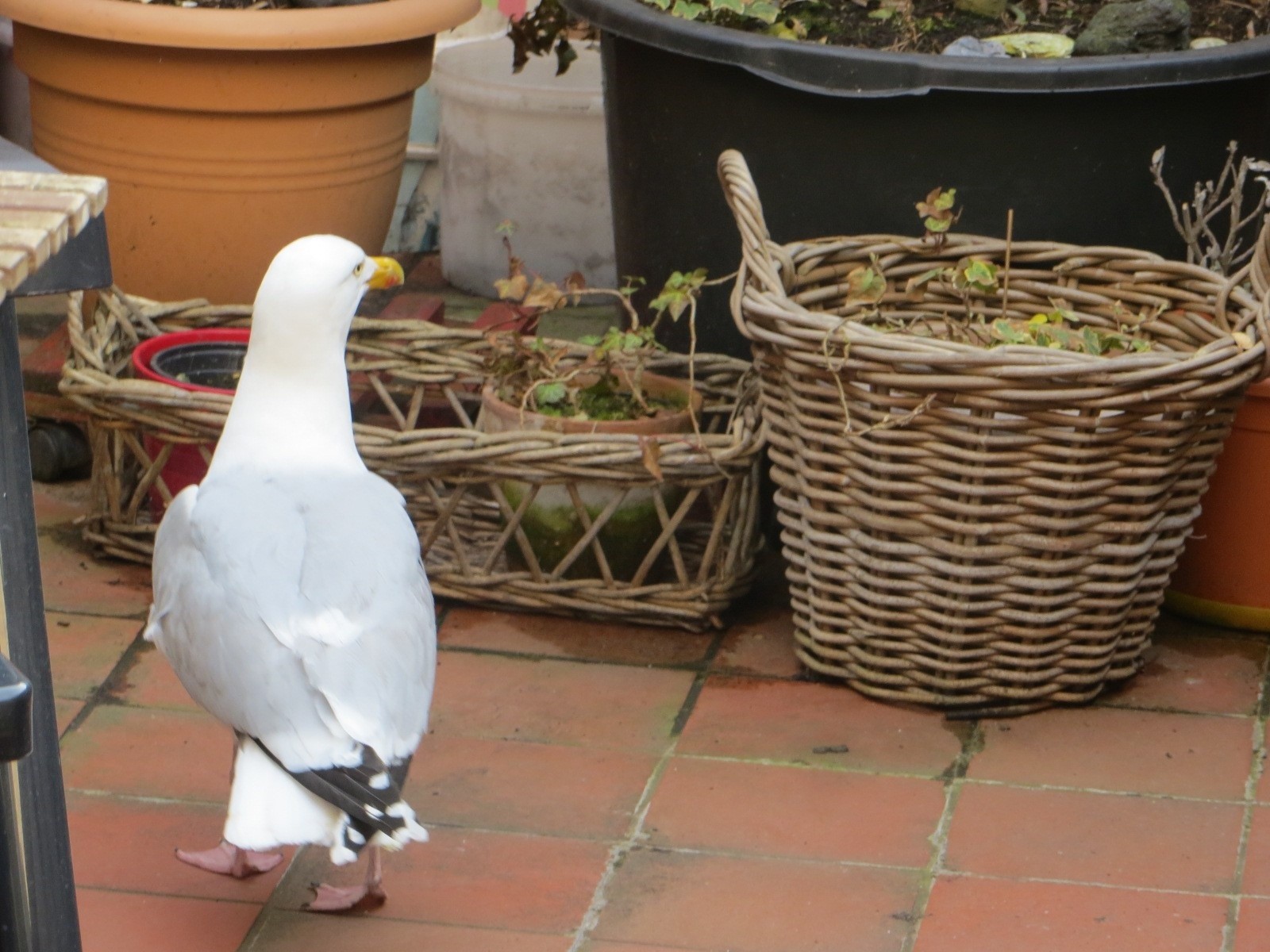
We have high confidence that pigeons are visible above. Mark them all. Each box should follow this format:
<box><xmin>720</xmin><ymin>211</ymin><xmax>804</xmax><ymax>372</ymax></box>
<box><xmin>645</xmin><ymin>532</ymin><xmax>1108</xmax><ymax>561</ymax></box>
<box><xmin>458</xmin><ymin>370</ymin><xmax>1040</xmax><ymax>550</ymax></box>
<box><xmin>144</xmin><ymin>234</ymin><xmax>438</xmax><ymax>913</ymax></box>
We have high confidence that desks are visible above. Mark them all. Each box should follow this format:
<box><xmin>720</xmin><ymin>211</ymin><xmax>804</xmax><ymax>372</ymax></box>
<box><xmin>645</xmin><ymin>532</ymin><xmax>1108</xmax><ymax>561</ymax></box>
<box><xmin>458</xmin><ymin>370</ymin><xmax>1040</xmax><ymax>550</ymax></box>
<box><xmin>0</xmin><ymin>174</ymin><xmax>112</xmax><ymax>952</ymax></box>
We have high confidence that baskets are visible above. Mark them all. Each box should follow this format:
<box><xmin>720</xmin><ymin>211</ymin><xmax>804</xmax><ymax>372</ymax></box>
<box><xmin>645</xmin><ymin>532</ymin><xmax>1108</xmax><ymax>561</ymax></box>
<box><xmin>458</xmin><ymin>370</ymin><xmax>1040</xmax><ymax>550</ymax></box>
<box><xmin>59</xmin><ymin>286</ymin><xmax>769</xmax><ymax>634</ymax></box>
<box><xmin>716</xmin><ymin>148</ymin><xmax>1270</xmax><ymax>719</ymax></box>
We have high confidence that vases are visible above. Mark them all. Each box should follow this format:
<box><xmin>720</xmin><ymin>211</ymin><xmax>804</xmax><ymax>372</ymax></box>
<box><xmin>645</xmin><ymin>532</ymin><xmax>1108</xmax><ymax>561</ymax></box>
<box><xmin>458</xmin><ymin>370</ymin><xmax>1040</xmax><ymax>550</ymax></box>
<box><xmin>134</xmin><ymin>328</ymin><xmax>253</xmax><ymax>525</ymax></box>
<box><xmin>0</xmin><ymin>0</ymin><xmax>481</xmax><ymax>318</ymax></box>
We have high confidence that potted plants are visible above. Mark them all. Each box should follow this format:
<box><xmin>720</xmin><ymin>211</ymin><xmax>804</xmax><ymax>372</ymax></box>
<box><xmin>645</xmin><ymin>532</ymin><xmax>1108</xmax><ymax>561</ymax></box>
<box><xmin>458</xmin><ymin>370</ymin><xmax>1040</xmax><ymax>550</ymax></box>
<box><xmin>509</xmin><ymin>0</ymin><xmax>1270</xmax><ymax>545</ymax></box>
<box><xmin>1147</xmin><ymin>138</ymin><xmax>1270</xmax><ymax>632</ymax></box>
<box><xmin>475</xmin><ymin>218</ymin><xmax>708</xmax><ymax>580</ymax></box>
